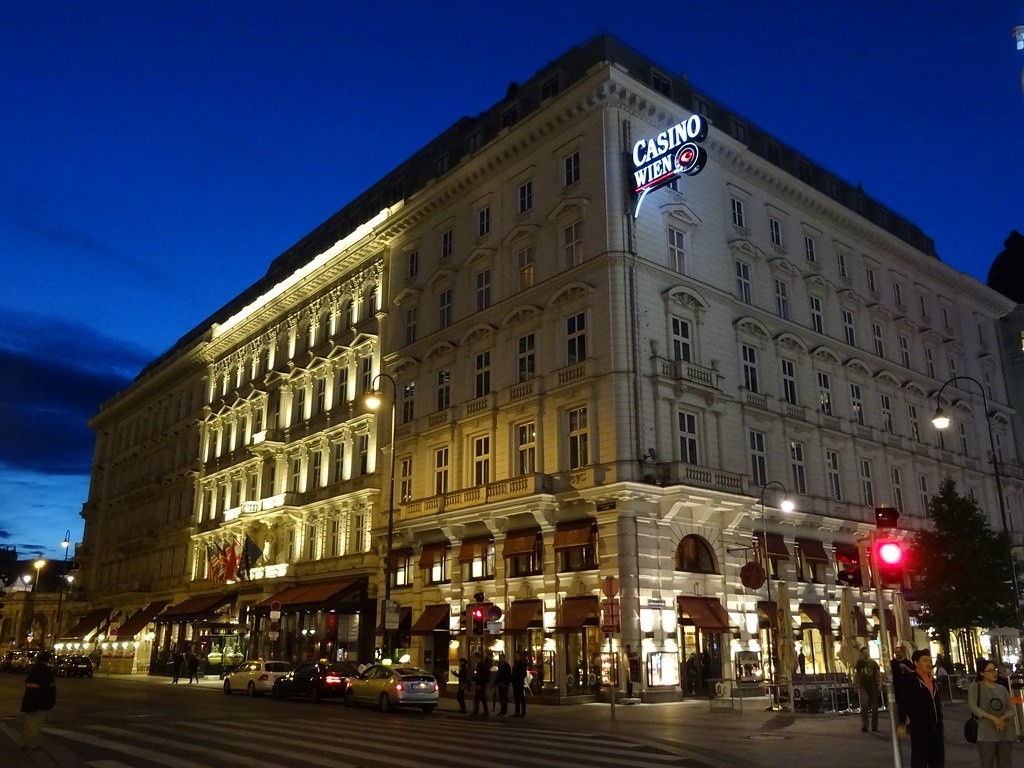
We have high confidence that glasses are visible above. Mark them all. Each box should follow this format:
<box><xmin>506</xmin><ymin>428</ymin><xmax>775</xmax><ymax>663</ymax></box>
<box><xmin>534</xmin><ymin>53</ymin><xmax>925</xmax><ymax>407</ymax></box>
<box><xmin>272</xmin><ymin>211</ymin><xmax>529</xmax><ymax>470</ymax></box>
<box><xmin>982</xmin><ymin>669</ymin><xmax>998</xmax><ymax>672</ymax></box>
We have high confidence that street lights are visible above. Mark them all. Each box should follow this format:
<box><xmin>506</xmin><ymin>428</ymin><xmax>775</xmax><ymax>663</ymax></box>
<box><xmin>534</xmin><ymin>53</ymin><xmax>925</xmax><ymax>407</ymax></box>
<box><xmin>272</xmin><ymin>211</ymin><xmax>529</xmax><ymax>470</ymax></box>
<box><xmin>930</xmin><ymin>376</ymin><xmax>1024</xmax><ymax>661</ymax></box>
<box><xmin>52</xmin><ymin>529</ymin><xmax>71</xmax><ymax>646</ymax></box>
<box><xmin>760</xmin><ymin>481</ymin><xmax>795</xmax><ymax>602</ymax></box>
<box><xmin>34</xmin><ymin>560</ymin><xmax>46</xmax><ymax>591</ymax></box>
<box><xmin>365</xmin><ymin>373</ymin><xmax>397</xmax><ymax>656</ymax></box>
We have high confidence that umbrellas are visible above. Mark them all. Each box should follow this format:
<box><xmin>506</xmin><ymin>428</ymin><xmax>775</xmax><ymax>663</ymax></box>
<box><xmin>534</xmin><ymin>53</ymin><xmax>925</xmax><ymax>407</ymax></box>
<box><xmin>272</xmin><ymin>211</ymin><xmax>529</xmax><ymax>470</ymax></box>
<box><xmin>776</xmin><ymin>583</ymin><xmax>797</xmax><ymax>714</ymax></box>
<box><xmin>838</xmin><ymin>587</ymin><xmax>919</xmax><ymax>710</ymax></box>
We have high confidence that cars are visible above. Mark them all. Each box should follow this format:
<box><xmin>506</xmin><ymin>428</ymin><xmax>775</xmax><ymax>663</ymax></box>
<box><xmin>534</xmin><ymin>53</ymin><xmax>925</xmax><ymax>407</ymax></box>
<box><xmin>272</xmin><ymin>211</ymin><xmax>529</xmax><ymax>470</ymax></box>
<box><xmin>345</xmin><ymin>664</ymin><xmax>440</xmax><ymax>713</ymax></box>
<box><xmin>0</xmin><ymin>650</ymin><xmax>94</xmax><ymax>679</ymax></box>
<box><xmin>224</xmin><ymin>660</ymin><xmax>294</xmax><ymax>697</ymax></box>
<box><xmin>273</xmin><ymin>661</ymin><xmax>362</xmax><ymax>703</ymax></box>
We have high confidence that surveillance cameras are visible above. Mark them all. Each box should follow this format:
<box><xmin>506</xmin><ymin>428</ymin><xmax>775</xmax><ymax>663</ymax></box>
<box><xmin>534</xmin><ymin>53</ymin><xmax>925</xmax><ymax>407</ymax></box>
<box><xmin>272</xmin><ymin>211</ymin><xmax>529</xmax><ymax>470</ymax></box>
<box><xmin>649</xmin><ymin>447</ymin><xmax>657</xmax><ymax>461</ymax></box>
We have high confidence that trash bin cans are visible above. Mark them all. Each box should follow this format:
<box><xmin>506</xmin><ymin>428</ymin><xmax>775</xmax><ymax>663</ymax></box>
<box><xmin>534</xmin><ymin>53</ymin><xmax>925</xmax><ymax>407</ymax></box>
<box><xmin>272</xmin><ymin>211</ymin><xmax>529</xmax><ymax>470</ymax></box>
<box><xmin>1007</xmin><ymin>673</ymin><xmax>1024</xmax><ymax>739</ymax></box>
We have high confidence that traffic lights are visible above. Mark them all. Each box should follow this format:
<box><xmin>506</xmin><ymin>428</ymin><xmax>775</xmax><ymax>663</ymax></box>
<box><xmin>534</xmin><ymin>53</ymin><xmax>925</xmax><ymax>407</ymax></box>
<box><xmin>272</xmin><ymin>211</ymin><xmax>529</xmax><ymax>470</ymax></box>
<box><xmin>461</xmin><ymin>603</ymin><xmax>472</xmax><ymax>637</ymax></box>
<box><xmin>876</xmin><ymin>506</ymin><xmax>902</xmax><ymax>565</ymax></box>
<box><xmin>473</xmin><ymin>608</ymin><xmax>484</xmax><ymax>636</ymax></box>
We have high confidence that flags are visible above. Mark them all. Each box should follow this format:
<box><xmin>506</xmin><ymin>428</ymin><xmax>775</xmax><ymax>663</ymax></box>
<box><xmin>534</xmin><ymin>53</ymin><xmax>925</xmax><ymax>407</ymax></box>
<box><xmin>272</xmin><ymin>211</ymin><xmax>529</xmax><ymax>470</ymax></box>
<box><xmin>207</xmin><ymin>536</ymin><xmax>262</xmax><ymax>581</ymax></box>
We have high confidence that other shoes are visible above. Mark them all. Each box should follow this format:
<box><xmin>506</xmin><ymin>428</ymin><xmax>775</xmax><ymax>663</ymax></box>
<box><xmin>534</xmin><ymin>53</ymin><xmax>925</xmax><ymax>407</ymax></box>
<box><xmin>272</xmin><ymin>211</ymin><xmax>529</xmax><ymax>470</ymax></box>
<box><xmin>23</xmin><ymin>742</ymin><xmax>42</xmax><ymax>752</ymax></box>
<box><xmin>872</xmin><ymin>727</ymin><xmax>878</xmax><ymax>731</ymax></box>
<box><xmin>172</xmin><ymin>680</ymin><xmax>199</xmax><ymax>685</ymax></box>
<box><xmin>861</xmin><ymin>726</ymin><xmax>868</xmax><ymax>732</ymax></box>
<box><xmin>459</xmin><ymin>708</ymin><xmax>526</xmax><ymax>718</ymax></box>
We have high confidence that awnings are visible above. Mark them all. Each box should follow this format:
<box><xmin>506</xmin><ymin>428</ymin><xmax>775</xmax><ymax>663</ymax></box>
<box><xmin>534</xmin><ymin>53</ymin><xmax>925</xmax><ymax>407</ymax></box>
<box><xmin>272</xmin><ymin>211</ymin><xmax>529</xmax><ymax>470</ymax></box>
<box><xmin>389</xmin><ymin>518</ymin><xmax>597</xmax><ymax>572</ymax></box>
<box><xmin>759</xmin><ymin>602</ymin><xmax>896</xmax><ymax>637</ymax></box>
<box><xmin>255</xmin><ymin>578</ymin><xmax>363</xmax><ymax>612</ymax></box>
<box><xmin>757</xmin><ymin>534</ymin><xmax>858</xmax><ymax>563</ymax></box>
<box><xmin>117</xmin><ymin>600</ymin><xmax>170</xmax><ymax>636</ymax></box>
<box><xmin>65</xmin><ymin>607</ymin><xmax>112</xmax><ymax>638</ymax></box>
<box><xmin>375</xmin><ymin>595</ymin><xmax>598</xmax><ymax>637</ymax></box>
<box><xmin>680</xmin><ymin>598</ymin><xmax>738</xmax><ymax>632</ymax></box>
<box><xmin>158</xmin><ymin>591</ymin><xmax>240</xmax><ymax>624</ymax></box>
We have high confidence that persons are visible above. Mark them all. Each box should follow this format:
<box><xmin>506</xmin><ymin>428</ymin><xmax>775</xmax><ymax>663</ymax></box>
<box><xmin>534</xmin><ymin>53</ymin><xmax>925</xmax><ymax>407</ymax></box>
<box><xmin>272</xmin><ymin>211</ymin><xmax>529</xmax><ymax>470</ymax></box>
<box><xmin>967</xmin><ymin>660</ymin><xmax>1015</xmax><ymax>768</ymax></box>
<box><xmin>897</xmin><ymin>649</ymin><xmax>944</xmax><ymax>768</ymax></box>
<box><xmin>932</xmin><ymin>654</ymin><xmax>944</xmax><ymax>675</ymax></box>
<box><xmin>450</xmin><ymin>652</ymin><xmax>527</xmax><ymax>717</ymax></box>
<box><xmin>189</xmin><ymin>655</ymin><xmax>199</xmax><ymax>683</ymax></box>
<box><xmin>976</xmin><ymin>652</ymin><xmax>993</xmax><ymax>662</ymax></box>
<box><xmin>22</xmin><ymin>652</ymin><xmax>56</xmax><ymax>749</ymax></box>
<box><xmin>855</xmin><ymin>647</ymin><xmax>882</xmax><ymax>732</ymax></box>
<box><xmin>890</xmin><ymin>647</ymin><xmax>915</xmax><ymax>688</ymax></box>
<box><xmin>173</xmin><ymin>651</ymin><xmax>184</xmax><ymax>684</ymax></box>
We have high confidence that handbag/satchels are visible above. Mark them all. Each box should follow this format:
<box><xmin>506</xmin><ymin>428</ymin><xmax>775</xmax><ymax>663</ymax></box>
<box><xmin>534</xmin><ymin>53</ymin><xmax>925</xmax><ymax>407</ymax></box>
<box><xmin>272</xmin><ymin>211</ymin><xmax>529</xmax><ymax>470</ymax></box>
<box><xmin>964</xmin><ymin>719</ymin><xmax>978</xmax><ymax>742</ymax></box>
